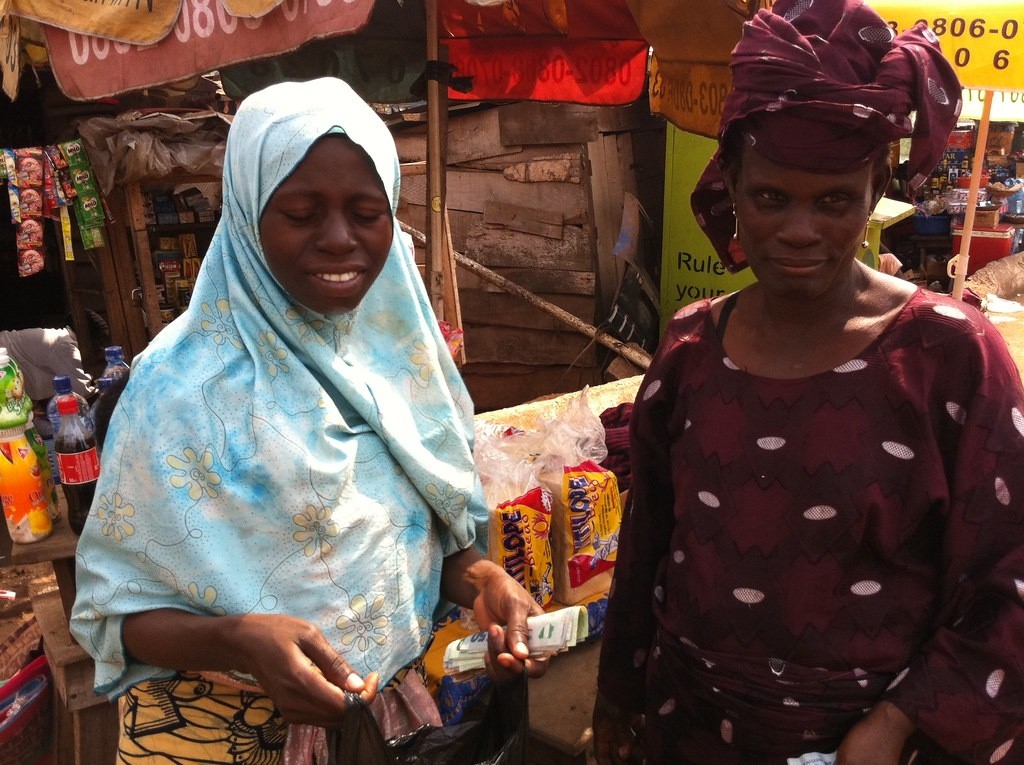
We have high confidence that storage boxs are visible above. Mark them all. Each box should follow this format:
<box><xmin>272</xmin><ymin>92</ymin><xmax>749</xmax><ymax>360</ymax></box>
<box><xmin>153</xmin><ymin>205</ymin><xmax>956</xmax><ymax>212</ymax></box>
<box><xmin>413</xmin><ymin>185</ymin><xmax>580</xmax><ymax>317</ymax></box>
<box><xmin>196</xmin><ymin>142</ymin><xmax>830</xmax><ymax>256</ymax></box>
<box><xmin>914</xmin><ymin>121</ymin><xmax>1024</xmax><ymax>275</ymax></box>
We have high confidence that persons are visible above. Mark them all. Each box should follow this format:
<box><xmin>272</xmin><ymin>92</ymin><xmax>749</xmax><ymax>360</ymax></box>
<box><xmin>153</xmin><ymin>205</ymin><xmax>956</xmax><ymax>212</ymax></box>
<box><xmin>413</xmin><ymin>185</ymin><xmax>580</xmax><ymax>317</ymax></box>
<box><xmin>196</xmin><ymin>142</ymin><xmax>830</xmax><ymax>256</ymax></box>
<box><xmin>69</xmin><ymin>77</ymin><xmax>550</xmax><ymax>765</ymax></box>
<box><xmin>592</xmin><ymin>1</ymin><xmax>1024</xmax><ymax>765</ymax></box>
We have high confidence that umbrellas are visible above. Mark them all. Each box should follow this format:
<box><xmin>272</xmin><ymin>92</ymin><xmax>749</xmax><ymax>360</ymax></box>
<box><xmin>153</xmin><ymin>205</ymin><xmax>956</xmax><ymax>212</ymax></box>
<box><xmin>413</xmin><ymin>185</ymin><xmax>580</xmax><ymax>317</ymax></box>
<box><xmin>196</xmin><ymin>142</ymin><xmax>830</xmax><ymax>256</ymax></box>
<box><xmin>43</xmin><ymin>0</ymin><xmax>752</xmax><ymax>323</ymax></box>
<box><xmin>865</xmin><ymin>0</ymin><xmax>1024</xmax><ymax>301</ymax></box>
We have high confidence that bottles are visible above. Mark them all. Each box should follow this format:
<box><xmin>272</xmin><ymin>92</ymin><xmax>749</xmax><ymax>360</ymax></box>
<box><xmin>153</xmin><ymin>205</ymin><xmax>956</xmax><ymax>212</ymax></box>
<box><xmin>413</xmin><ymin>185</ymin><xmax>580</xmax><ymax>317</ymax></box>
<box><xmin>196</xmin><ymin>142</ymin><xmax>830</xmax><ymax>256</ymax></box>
<box><xmin>0</xmin><ymin>347</ymin><xmax>61</xmax><ymax>545</ymax></box>
<box><xmin>47</xmin><ymin>375</ymin><xmax>94</xmax><ymax>447</ymax></box>
<box><xmin>102</xmin><ymin>345</ymin><xmax>130</xmax><ymax>380</ymax></box>
<box><xmin>90</xmin><ymin>377</ymin><xmax>113</xmax><ymax>432</ymax></box>
<box><xmin>55</xmin><ymin>396</ymin><xmax>100</xmax><ymax>535</ymax></box>
